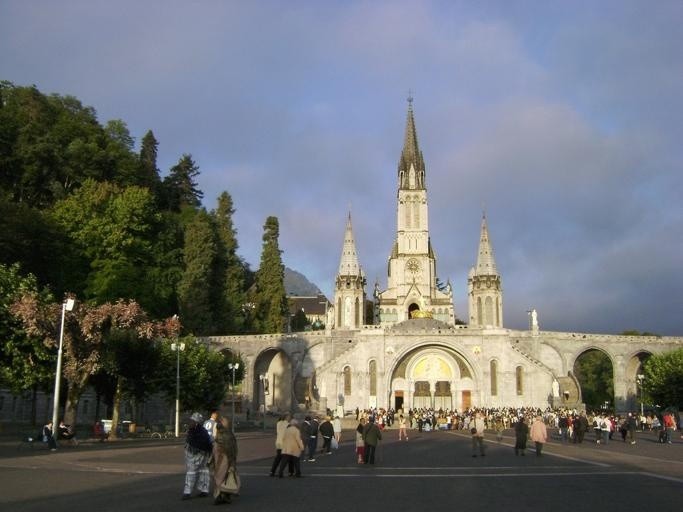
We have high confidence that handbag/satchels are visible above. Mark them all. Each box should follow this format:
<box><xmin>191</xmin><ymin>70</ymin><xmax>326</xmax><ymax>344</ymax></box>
<box><xmin>329</xmin><ymin>436</ymin><xmax>339</xmax><ymax>450</ymax></box>
<box><xmin>186</xmin><ymin>422</ymin><xmax>210</xmax><ymax>453</ymax></box>
<box><xmin>42</xmin><ymin>435</ymin><xmax>48</xmax><ymax>442</ymax></box>
<box><xmin>219</xmin><ymin>466</ymin><xmax>241</xmax><ymax>495</ymax></box>
<box><xmin>470</xmin><ymin>427</ymin><xmax>477</xmax><ymax>434</ymax></box>
<box><xmin>558</xmin><ymin>427</ymin><xmax>562</xmax><ymax>435</ymax></box>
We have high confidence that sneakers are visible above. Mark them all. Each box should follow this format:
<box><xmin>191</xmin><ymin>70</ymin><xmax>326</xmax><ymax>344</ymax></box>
<box><xmin>596</xmin><ymin>439</ymin><xmax>601</xmax><ymax>445</ymax></box>
<box><xmin>299</xmin><ymin>458</ymin><xmax>316</xmax><ymax>462</ymax></box>
<box><xmin>659</xmin><ymin>439</ymin><xmax>673</xmax><ymax>445</ymax></box>
<box><xmin>471</xmin><ymin>453</ymin><xmax>486</xmax><ymax>457</ymax></box>
<box><xmin>49</xmin><ymin>447</ymin><xmax>58</xmax><ymax>452</ymax></box>
<box><xmin>214</xmin><ymin>495</ymin><xmax>231</xmax><ymax>506</ymax></box>
<box><xmin>319</xmin><ymin>452</ymin><xmax>332</xmax><ymax>456</ymax></box>
<box><xmin>398</xmin><ymin>436</ymin><xmax>409</xmax><ymax>442</ymax></box>
<box><xmin>631</xmin><ymin>440</ymin><xmax>637</xmax><ymax>445</ymax></box>
<box><xmin>182</xmin><ymin>491</ymin><xmax>209</xmax><ymax>501</ymax></box>
<box><xmin>515</xmin><ymin>451</ymin><xmax>542</xmax><ymax>457</ymax></box>
<box><xmin>269</xmin><ymin>469</ymin><xmax>301</xmax><ymax>479</ymax></box>
<box><xmin>356</xmin><ymin>460</ymin><xmax>374</xmax><ymax>465</ymax></box>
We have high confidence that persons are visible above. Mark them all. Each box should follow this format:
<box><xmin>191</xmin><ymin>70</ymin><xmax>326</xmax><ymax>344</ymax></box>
<box><xmin>355</xmin><ymin>406</ymin><xmax>547</xmax><ymax>466</ymax></box>
<box><xmin>183</xmin><ymin>411</ymin><xmax>241</xmax><ymax>505</ymax></box>
<box><xmin>59</xmin><ymin>420</ymin><xmax>79</xmax><ymax>448</ymax></box>
<box><xmin>269</xmin><ymin>413</ymin><xmax>341</xmax><ymax>478</ymax></box>
<box><xmin>543</xmin><ymin>407</ymin><xmax>677</xmax><ymax>445</ymax></box>
<box><xmin>43</xmin><ymin>420</ymin><xmax>58</xmax><ymax>451</ymax></box>
<box><xmin>94</xmin><ymin>421</ymin><xmax>109</xmax><ymax>443</ymax></box>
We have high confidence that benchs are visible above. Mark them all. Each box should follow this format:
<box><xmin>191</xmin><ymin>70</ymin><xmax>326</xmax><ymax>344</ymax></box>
<box><xmin>73</xmin><ymin>424</ymin><xmax>111</xmax><ymax>450</ymax></box>
<box><xmin>17</xmin><ymin>427</ymin><xmax>78</xmax><ymax>451</ymax></box>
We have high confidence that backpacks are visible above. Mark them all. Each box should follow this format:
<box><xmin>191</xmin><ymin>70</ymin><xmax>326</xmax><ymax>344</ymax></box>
<box><xmin>591</xmin><ymin>420</ymin><xmax>608</xmax><ymax>428</ymax></box>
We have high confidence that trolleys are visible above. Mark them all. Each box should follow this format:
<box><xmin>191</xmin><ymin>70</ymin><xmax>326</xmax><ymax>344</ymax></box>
<box><xmin>656</xmin><ymin>425</ymin><xmax>667</xmax><ymax>445</ymax></box>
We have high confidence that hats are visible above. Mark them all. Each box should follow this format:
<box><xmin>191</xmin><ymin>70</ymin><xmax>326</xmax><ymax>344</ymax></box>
<box><xmin>189</xmin><ymin>411</ymin><xmax>203</xmax><ymax>424</ymax></box>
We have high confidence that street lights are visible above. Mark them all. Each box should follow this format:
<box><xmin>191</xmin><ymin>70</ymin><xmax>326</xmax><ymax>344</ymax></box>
<box><xmin>49</xmin><ymin>293</ymin><xmax>76</xmax><ymax>443</ymax></box>
<box><xmin>172</xmin><ymin>337</ymin><xmax>187</xmax><ymax>439</ymax></box>
<box><xmin>229</xmin><ymin>354</ymin><xmax>240</xmax><ymax>434</ymax></box>
<box><xmin>259</xmin><ymin>372</ymin><xmax>269</xmax><ymax>434</ymax></box>
<box><xmin>637</xmin><ymin>373</ymin><xmax>645</xmax><ymax>416</ymax></box>
